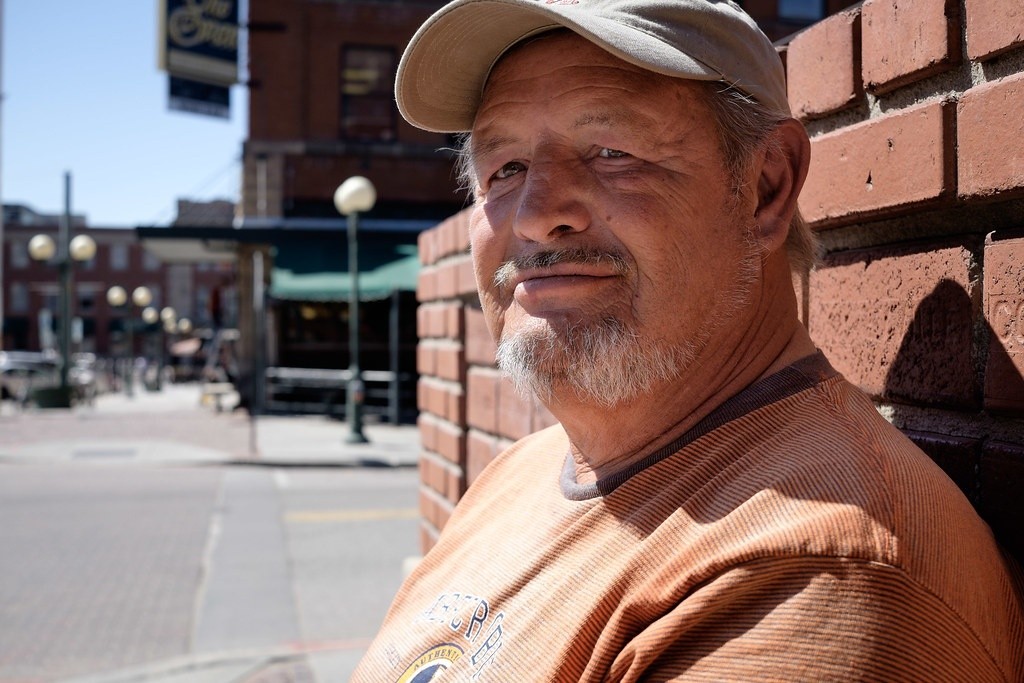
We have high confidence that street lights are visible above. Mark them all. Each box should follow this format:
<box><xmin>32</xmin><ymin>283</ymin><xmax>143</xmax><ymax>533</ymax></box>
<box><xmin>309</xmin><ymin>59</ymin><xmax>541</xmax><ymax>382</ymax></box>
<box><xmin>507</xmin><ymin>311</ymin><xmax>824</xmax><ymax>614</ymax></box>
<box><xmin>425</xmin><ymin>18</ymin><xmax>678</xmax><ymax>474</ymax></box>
<box><xmin>334</xmin><ymin>176</ymin><xmax>378</xmax><ymax>443</ymax></box>
<box><xmin>26</xmin><ymin>233</ymin><xmax>96</xmax><ymax>406</ymax></box>
<box><xmin>107</xmin><ymin>286</ymin><xmax>192</xmax><ymax>392</ymax></box>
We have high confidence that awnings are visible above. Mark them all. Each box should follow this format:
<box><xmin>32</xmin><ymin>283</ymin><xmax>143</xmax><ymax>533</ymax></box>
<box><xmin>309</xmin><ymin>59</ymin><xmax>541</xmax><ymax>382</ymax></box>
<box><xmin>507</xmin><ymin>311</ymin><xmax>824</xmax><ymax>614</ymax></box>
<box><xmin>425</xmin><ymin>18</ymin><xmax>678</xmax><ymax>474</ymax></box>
<box><xmin>270</xmin><ymin>245</ymin><xmax>418</xmax><ymax>304</ymax></box>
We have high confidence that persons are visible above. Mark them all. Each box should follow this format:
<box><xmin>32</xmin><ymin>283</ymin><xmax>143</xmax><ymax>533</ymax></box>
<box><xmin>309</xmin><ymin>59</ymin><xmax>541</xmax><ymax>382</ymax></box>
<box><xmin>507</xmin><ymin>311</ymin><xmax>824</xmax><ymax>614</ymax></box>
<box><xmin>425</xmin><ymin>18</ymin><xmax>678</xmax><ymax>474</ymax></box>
<box><xmin>349</xmin><ymin>0</ymin><xmax>1024</xmax><ymax>683</ymax></box>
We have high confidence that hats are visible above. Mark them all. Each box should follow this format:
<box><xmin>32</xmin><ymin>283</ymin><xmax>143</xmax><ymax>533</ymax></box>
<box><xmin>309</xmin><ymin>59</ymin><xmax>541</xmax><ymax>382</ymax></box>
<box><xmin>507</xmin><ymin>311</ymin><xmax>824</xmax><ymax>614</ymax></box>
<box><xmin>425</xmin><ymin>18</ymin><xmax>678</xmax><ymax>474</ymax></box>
<box><xmin>394</xmin><ymin>1</ymin><xmax>795</xmax><ymax>134</ymax></box>
